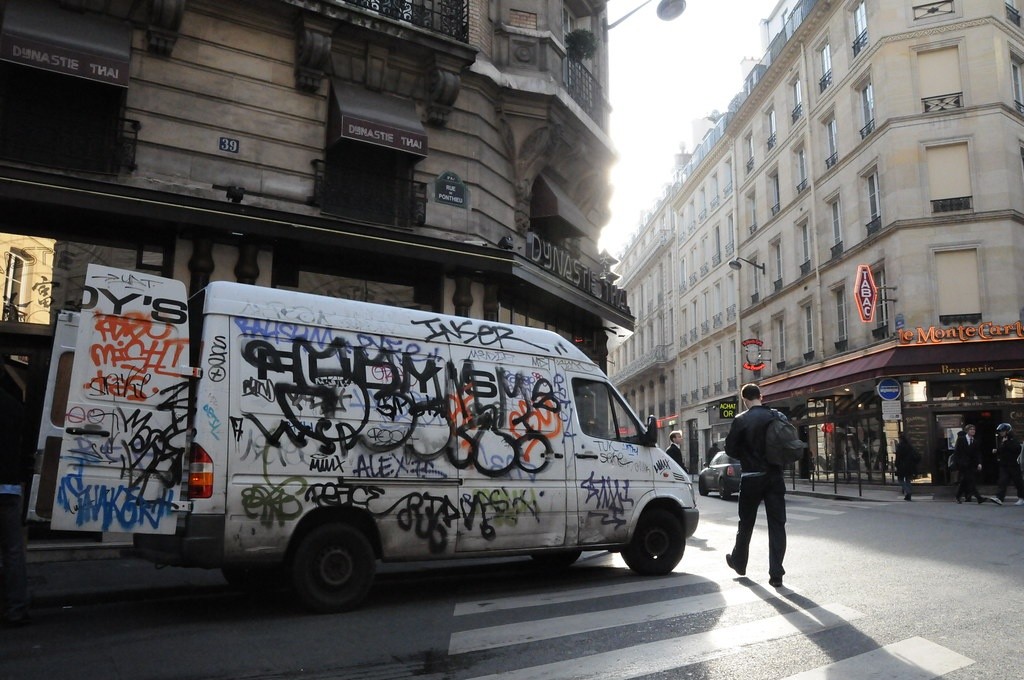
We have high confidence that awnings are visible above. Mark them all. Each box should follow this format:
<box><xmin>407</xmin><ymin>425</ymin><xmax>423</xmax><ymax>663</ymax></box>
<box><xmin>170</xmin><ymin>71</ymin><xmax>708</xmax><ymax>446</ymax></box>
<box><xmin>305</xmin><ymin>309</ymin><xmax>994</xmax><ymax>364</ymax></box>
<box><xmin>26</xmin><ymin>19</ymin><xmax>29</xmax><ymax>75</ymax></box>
<box><xmin>326</xmin><ymin>76</ymin><xmax>429</xmax><ymax>168</ymax></box>
<box><xmin>0</xmin><ymin>0</ymin><xmax>130</xmax><ymax>88</ymax></box>
<box><xmin>530</xmin><ymin>171</ymin><xmax>597</xmax><ymax>244</ymax></box>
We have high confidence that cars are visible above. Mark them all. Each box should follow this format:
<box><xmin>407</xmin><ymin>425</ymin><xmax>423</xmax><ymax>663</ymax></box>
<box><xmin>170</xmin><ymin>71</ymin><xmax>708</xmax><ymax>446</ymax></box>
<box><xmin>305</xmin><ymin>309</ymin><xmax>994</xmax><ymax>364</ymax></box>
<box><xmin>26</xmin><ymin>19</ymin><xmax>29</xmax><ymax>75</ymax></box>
<box><xmin>698</xmin><ymin>450</ymin><xmax>745</xmax><ymax>501</ymax></box>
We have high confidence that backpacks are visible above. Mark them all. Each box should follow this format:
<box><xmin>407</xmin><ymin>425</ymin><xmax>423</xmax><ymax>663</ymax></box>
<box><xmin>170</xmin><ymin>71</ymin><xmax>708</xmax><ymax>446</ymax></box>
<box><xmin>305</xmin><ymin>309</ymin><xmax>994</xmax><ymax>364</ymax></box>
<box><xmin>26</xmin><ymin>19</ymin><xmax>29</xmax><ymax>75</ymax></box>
<box><xmin>762</xmin><ymin>409</ymin><xmax>803</xmax><ymax>465</ymax></box>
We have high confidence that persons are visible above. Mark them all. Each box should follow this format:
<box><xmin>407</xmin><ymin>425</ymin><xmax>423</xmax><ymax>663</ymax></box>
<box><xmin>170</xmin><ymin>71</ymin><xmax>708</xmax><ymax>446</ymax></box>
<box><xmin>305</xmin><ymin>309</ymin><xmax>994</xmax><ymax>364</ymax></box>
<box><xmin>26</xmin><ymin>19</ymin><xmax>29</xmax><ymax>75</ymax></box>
<box><xmin>992</xmin><ymin>423</ymin><xmax>1024</xmax><ymax>506</ymax></box>
<box><xmin>704</xmin><ymin>443</ymin><xmax>721</xmax><ymax>468</ymax></box>
<box><xmin>953</xmin><ymin>423</ymin><xmax>988</xmax><ymax>505</ymax></box>
<box><xmin>666</xmin><ymin>430</ymin><xmax>689</xmax><ymax>475</ymax></box>
<box><xmin>895</xmin><ymin>435</ymin><xmax>914</xmax><ymax>501</ymax></box>
<box><xmin>724</xmin><ymin>386</ymin><xmax>789</xmax><ymax>588</ymax></box>
<box><xmin>0</xmin><ymin>376</ymin><xmax>42</xmax><ymax>628</ymax></box>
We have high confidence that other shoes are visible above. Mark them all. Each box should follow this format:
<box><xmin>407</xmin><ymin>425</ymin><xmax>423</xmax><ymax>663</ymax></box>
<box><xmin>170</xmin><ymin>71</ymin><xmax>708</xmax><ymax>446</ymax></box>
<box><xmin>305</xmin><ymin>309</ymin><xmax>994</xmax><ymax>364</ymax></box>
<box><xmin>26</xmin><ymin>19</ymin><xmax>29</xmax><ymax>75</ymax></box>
<box><xmin>977</xmin><ymin>496</ymin><xmax>988</xmax><ymax>504</ymax></box>
<box><xmin>726</xmin><ymin>554</ymin><xmax>746</xmax><ymax>575</ymax></box>
<box><xmin>990</xmin><ymin>497</ymin><xmax>1002</xmax><ymax>505</ymax></box>
<box><xmin>904</xmin><ymin>493</ymin><xmax>912</xmax><ymax>500</ymax></box>
<box><xmin>769</xmin><ymin>574</ymin><xmax>782</xmax><ymax>586</ymax></box>
<box><xmin>964</xmin><ymin>499</ymin><xmax>971</xmax><ymax>502</ymax></box>
<box><xmin>956</xmin><ymin>496</ymin><xmax>962</xmax><ymax>504</ymax></box>
<box><xmin>1015</xmin><ymin>498</ymin><xmax>1024</xmax><ymax>506</ymax></box>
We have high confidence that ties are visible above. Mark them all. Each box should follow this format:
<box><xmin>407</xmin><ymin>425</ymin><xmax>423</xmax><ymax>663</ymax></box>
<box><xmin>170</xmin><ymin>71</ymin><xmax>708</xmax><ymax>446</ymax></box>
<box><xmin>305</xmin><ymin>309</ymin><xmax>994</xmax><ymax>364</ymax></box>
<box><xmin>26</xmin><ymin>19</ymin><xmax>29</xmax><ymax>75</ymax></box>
<box><xmin>970</xmin><ymin>439</ymin><xmax>972</xmax><ymax>445</ymax></box>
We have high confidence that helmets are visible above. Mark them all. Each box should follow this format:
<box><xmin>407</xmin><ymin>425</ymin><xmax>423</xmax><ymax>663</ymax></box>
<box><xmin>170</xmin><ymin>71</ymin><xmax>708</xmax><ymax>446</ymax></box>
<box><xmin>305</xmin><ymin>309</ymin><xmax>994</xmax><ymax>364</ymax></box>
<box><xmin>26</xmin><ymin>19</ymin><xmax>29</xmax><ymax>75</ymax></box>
<box><xmin>997</xmin><ymin>423</ymin><xmax>1011</xmax><ymax>433</ymax></box>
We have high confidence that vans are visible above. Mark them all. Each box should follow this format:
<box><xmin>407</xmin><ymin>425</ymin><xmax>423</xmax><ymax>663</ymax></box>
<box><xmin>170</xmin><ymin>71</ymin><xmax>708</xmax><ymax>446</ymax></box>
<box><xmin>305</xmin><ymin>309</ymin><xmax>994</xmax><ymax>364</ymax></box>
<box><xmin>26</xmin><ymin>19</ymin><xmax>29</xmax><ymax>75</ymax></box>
<box><xmin>25</xmin><ymin>262</ymin><xmax>699</xmax><ymax>615</ymax></box>
